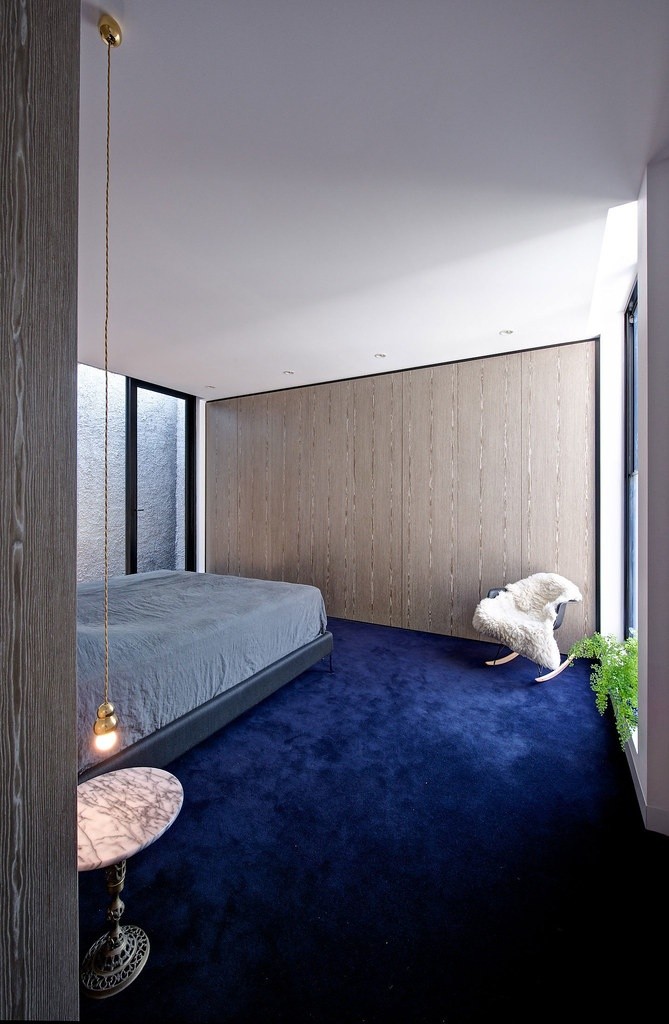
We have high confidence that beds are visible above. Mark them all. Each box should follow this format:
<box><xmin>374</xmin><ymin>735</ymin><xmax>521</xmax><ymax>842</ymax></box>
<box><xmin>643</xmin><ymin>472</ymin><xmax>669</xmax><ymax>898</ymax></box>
<box><xmin>76</xmin><ymin>569</ymin><xmax>336</xmax><ymax>783</ymax></box>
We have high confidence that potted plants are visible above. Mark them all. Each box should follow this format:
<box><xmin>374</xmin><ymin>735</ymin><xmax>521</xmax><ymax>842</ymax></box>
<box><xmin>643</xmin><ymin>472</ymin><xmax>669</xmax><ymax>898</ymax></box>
<box><xmin>567</xmin><ymin>631</ymin><xmax>639</xmax><ymax>753</ymax></box>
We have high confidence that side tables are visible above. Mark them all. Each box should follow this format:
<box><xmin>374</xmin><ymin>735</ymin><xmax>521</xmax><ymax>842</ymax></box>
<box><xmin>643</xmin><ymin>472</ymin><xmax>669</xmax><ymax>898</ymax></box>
<box><xmin>77</xmin><ymin>765</ymin><xmax>184</xmax><ymax>1000</ymax></box>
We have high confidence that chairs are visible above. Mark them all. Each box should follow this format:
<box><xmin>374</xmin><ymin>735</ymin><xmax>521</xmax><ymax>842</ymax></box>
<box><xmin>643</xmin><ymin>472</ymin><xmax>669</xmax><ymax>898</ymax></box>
<box><xmin>485</xmin><ymin>587</ymin><xmax>580</xmax><ymax>682</ymax></box>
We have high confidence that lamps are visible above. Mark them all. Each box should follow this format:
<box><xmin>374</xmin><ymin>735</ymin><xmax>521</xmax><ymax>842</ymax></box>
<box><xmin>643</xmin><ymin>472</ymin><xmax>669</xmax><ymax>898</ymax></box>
<box><xmin>93</xmin><ymin>13</ymin><xmax>122</xmax><ymax>763</ymax></box>
